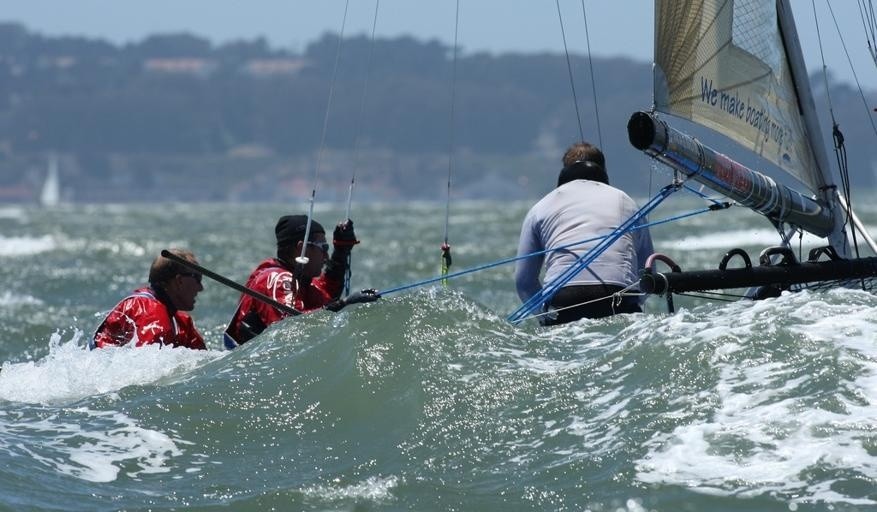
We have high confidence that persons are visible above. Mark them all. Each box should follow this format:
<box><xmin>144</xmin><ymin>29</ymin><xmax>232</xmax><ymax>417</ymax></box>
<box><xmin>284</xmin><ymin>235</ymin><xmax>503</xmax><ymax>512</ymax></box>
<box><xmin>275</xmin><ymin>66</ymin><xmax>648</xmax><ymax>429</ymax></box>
<box><xmin>512</xmin><ymin>140</ymin><xmax>658</xmax><ymax>332</ymax></box>
<box><xmin>82</xmin><ymin>247</ymin><xmax>208</xmax><ymax>355</ymax></box>
<box><xmin>220</xmin><ymin>213</ymin><xmax>382</xmax><ymax>349</ymax></box>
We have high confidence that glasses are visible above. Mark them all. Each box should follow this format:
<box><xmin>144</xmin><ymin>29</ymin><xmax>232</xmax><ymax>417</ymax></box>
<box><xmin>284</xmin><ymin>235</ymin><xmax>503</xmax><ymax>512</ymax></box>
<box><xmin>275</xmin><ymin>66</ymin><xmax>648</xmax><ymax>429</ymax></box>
<box><xmin>176</xmin><ymin>270</ymin><xmax>202</xmax><ymax>284</ymax></box>
<box><xmin>302</xmin><ymin>238</ymin><xmax>328</xmax><ymax>252</ymax></box>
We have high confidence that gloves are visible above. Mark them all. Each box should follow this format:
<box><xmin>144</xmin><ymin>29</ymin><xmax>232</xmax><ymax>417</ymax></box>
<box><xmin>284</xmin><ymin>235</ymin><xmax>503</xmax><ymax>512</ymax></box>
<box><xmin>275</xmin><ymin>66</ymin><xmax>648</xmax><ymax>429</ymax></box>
<box><xmin>341</xmin><ymin>287</ymin><xmax>379</xmax><ymax>308</ymax></box>
<box><xmin>331</xmin><ymin>219</ymin><xmax>361</xmax><ymax>257</ymax></box>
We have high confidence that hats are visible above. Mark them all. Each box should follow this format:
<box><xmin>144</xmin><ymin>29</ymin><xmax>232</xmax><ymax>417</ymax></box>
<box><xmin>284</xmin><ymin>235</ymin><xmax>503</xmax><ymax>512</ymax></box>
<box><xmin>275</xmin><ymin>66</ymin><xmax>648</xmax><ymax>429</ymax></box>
<box><xmin>273</xmin><ymin>213</ymin><xmax>328</xmax><ymax>258</ymax></box>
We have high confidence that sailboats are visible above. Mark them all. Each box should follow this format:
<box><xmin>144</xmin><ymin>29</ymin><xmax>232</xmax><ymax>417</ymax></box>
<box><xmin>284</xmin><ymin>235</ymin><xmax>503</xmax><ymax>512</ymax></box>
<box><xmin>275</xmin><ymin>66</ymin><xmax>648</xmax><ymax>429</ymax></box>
<box><xmin>288</xmin><ymin>0</ymin><xmax>877</xmax><ymax>326</ymax></box>
